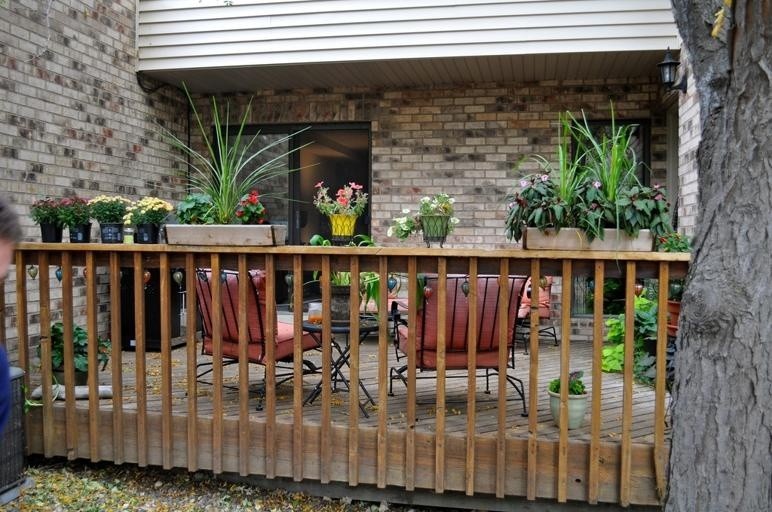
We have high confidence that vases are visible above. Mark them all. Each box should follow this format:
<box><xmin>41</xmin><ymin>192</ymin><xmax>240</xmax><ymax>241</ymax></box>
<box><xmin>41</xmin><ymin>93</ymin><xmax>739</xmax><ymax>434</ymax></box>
<box><xmin>40</xmin><ymin>221</ymin><xmax>292</xmax><ymax>247</ymax></box>
<box><xmin>546</xmin><ymin>390</ymin><xmax>589</xmax><ymax>430</ymax></box>
<box><xmin>418</xmin><ymin>213</ymin><xmax>453</xmax><ymax>249</ymax></box>
<box><xmin>329</xmin><ymin>211</ymin><xmax>359</xmax><ymax>246</ymax></box>
<box><xmin>521</xmin><ymin>223</ymin><xmax>655</xmax><ymax>252</ymax></box>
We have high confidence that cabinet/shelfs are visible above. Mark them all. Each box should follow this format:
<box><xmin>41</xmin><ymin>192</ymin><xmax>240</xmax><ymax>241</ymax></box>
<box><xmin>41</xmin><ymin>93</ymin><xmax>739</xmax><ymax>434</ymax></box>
<box><xmin>0</xmin><ymin>364</ymin><xmax>33</xmax><ymax>506</ymax></box>
<box><xmin>110</xmin><ymin>269</ymin><xmax>193</xmax><ymax>353</ymax></box>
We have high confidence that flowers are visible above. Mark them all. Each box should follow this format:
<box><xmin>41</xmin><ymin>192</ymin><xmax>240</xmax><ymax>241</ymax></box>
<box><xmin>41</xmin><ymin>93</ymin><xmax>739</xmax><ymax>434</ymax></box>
<box><xmin>310</xmin><ymin>177</ymin><xmax>369</xmax><ymax>216</ymax></box>
<box><xmin>55</xmin><ymin>197</ymin><xmax>90</xmax><ymax>220</ymax></box>
<box><xmin>123</xmin><ymin>196</ymin><xmax>173</xmax><ymax>221</ymax></box>
<box><xmin>504</xmin><ymin>112</ymin><xmax>669</xmax><ymax>230</ymax></box>
<box><xmin>385</xmin><ymin>190</ymin><xmax>462</xmax><ymax>239</ymax></box>
<box><xmin>234</xmin><ymin>191</ymin><xmax>266</xmax><ymax>221</ymax></box>
<box><xmin>549</xmin><ymin>371</ymin><xmax>590</xmax><ymax>395</ymax></box>
<box><xmin>31</xmin><ymin>197</ymin><xmax>63</xmax><ymax>221</ymax></box>
<box><xmin>86</xmin><ymin>195</ymin><xmax>131</xmax><ymax>221</ymax></box>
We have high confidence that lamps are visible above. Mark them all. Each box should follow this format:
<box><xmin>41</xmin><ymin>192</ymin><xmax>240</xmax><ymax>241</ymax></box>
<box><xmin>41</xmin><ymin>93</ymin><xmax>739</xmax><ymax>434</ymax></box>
<box><xmin>657</xmin><ymin>46</ymin><xmax>690</xmax><ymax>100</ymax></box>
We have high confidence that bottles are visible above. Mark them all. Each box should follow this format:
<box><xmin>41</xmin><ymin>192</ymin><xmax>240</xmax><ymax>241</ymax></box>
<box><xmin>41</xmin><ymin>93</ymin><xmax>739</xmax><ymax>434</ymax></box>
<box><xmin>157</xmin><ymin>228</ymin><xmax>166</xmax><ymax>244</ymax></box>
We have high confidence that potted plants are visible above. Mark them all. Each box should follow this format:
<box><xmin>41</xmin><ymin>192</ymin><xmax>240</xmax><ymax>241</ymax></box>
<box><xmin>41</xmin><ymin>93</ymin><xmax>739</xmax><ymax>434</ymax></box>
<box><xmin>665</xmin><ymin>277</ymin><xmax>685</xmax><ymax>333</ymax></box>
<box><xmin>37</xmin><ymin>319</ymin><xmax>112</xmax><ymax>387</ymax></box>
<box><xmin>307</xmin><ymin>233</ymin><xmax>377</xmax><ymax>322</ymax></box>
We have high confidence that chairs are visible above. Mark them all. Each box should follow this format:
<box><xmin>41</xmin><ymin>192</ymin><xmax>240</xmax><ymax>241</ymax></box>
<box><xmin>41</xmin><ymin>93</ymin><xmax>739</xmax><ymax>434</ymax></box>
<box><xmin>512</xmin><ymin>274</ymin><xmax>557</xmax><ymax>357</ymax></box>
<box><xmin>191</xmin><ymin>267</ymin><xmax>336</xmax><ymax>414</ymax></box>
<box><xmin>385</xmin><ymin>274</ymin><xmax>530</xmax><ymax>419</ymax></box>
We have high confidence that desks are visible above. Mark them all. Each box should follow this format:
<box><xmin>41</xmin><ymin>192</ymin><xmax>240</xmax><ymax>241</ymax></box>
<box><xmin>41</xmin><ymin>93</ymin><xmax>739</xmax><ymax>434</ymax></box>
<box><xmin>298</xmin><ymin>319</ymin><xmax>384</xmax><ymax>419</ymax></box>
<box><xmin>352</xmin><ymin>296</ymin><xmax>414</xmax><ymax>363</ymax></box>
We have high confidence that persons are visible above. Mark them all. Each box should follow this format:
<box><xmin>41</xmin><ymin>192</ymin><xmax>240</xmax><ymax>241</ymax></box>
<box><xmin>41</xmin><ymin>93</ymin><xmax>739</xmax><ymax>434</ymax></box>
<box><xmin>1</xmin><ymin>200</ymin><xmax>24</xmax><ymax>430</ymax></box>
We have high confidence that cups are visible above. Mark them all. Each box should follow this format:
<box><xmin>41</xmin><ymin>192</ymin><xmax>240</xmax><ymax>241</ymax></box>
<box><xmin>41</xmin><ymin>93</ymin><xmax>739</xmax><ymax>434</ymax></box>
<box><xmin>124</xmin><ymin>227</ymin><xmax>134</xmax><ymax>243</ymax></box>
<box><xmin>308</xmin><ymin>302</ymin><xmax>322</xmax><ymax>324</ymax></box>
